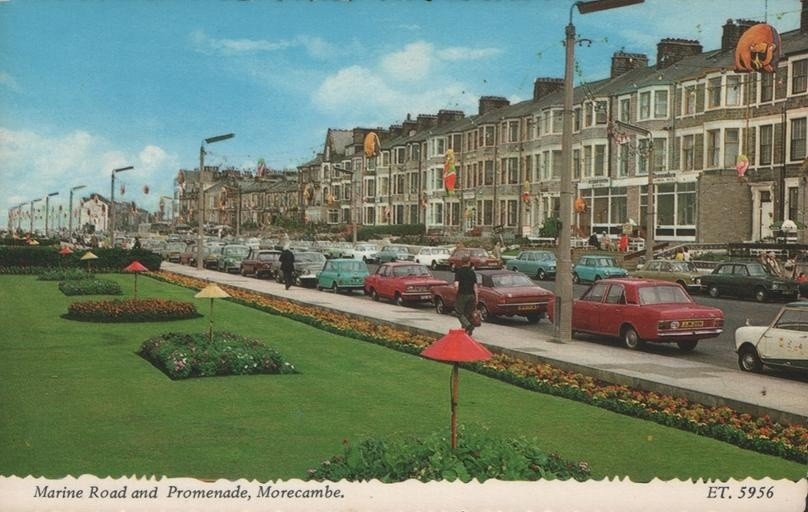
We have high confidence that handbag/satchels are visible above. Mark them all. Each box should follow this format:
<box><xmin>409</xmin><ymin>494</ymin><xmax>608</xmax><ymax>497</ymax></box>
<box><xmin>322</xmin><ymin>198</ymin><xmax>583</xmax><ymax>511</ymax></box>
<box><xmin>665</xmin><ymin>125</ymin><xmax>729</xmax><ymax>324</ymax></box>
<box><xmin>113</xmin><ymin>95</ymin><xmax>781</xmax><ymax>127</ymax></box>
<box><xmin>471</xmin><ymin>310</ymin><xmax>481</xmax><ymax>327</ymax></box>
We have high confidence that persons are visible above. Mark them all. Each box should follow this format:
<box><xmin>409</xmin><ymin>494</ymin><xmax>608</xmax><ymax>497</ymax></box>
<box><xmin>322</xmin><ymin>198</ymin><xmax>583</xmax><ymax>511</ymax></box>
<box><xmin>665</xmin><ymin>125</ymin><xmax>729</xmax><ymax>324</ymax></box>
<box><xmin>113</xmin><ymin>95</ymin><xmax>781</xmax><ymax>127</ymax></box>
<box><xmin>683</xmin><ymin>247</ymin><xmax>690</xmax><ymax>262</ymax></box>
<box><xmin>674</xmin><ymin>247</ymin><xmax>682</xmax><ymax>261</ymax></box>
<box><xmin>795</xmin><ymin>273</ymin><xmax>808</xmax><ymax>283</ymax></box>
<box><xmin>618</xmin><ymin>233</ymin><xmax>628</xmax><ymax>252</ymax></box>
<box><xmin>589</xmin><ymin>231</ymin><xmax>607</xmax><ymax>251</ymax></box>
<box><xmin>766</xmin><ymin>251</ymin><xmax>780</xmax><ymax>276</ymax></box>
<box><xmin>279</xmin><ymin>244</ymin><xmax>296</xmax><ymax>290</ymax></box>
<box><xmin>783</xmin><ymin>255</ymin><xmax>797</xmax><ymax>278</ymax></box>
<box><xmin>70</xmin><ymin>228</ymin><xmax>144</xmax><ymax>250</ymax></box>
<box><xmin>602</xmin><ymin>229</ymin><xmax>613</xmax><ymax>251</ymax></box>
<box><xmin>453</xmin><ymin>256</ymin><xmax>478</xmax><ymax>336</ymax></box>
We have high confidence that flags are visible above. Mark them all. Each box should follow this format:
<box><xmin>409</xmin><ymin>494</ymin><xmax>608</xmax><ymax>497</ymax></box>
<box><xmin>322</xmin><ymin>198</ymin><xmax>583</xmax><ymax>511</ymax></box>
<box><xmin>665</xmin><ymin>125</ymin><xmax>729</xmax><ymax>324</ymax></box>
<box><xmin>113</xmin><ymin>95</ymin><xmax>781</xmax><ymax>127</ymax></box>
<box><xmin>443</xmin><ymin>147</ymin><xmax>458</xmax><ymax>196</ymax></box>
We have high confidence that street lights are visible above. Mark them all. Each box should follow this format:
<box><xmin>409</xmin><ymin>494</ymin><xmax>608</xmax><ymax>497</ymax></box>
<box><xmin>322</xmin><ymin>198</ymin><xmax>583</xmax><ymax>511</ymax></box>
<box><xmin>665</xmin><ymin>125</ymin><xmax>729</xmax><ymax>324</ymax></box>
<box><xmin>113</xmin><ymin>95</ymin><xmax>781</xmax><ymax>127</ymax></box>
<box><xmin>8</xmin><ymin>199</ymin><xmax>42</xmax><ymax>238</ymax></box>
<box><xmin>552</xmin><ymin>0</ymin><xmax>644</xmax><ymax>346</ymax></box>
<box><xmin>108</xmin><ymin>164</ymin><xmax>133</xmax><ymax>250</ymax></box>
<box><xmin>195</xmin><ymin>132</ymin><xmax>234</xmax><ymax>269</ymax></box>
<box><xmin>69</xmin><ymin>185</ymin><xmax>87</xmax><ymax>244</ymax></box>
<box><xmin>45</xmin><ymin>192</ymin><xmax>59</xmax><ymax>239</ymax></box>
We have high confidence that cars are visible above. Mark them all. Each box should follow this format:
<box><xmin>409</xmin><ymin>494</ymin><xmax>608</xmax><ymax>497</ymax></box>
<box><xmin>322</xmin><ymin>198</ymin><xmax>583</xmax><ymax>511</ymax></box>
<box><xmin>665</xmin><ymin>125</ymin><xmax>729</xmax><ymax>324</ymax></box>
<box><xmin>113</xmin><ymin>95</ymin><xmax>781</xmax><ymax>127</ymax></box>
<box><xmin>571</xmin><ymin>254</ymin><xmax>626</xmax><ymax>283</ymax></box>
<box><xmin>733</xmin><ymin>302</ymin><xmax>807</xmax><ymax>377</ymax></box>
<box><xmin>631</xmin><ymin>259</ymin><xmax>706</xmax><ymax>295</ymax></box>
<box><xmin>505</xmin><ymin>250</ymin><xmax>556</xmax><ymax>279</ymax></box>
<box><xmin>375</xmin><ymin>243</ymin><xmax>414</xmax><ymax>265</ymax></box>
<box><xmin>547</xmin><ymin>279</ymin><xmax>724</xmax><ymax>353</ymax></box>
<box><xmin>697</xmin><ymin>262</ymin><xmax>799</xmax><ymax>308</ymax></box>
<box><xmin>54</xmin><ymin>223</ymin><xmax>391</xmax><ymax>286</ymax></box>
<box><xmin>413</xmin><ymin>247</ymin><xmax>451</xmax><ymax>268</ymax></box>
<box><xmin>447</xmin><ymin>248</ymin><xmax>502</xmax><ymax>271</ymax></box>
<box><xmin>429</xmin><ymin>269</ymin><xmax>552</xmax><ymax>324</ymax></box>
<box><xmin>315</xmin><ymin>258</ymin><xmax>370</xmax><ymax>293</ymax></box>
<box><xmin>364</xmin><ymin>262</ymin><xmax>448</xmax><ymax>306</ymax></box>
